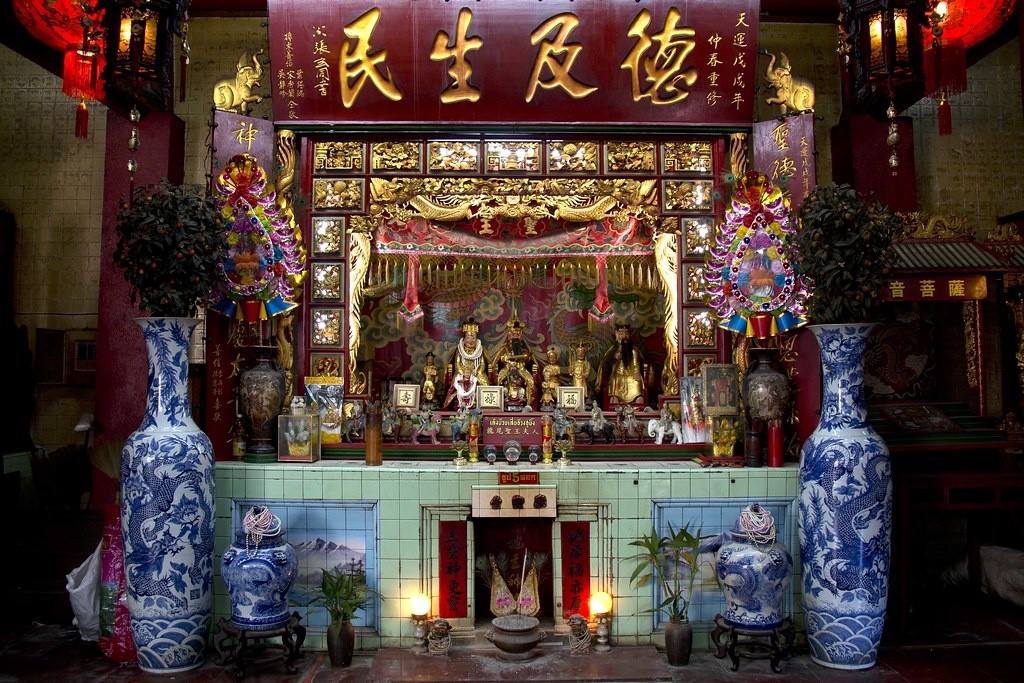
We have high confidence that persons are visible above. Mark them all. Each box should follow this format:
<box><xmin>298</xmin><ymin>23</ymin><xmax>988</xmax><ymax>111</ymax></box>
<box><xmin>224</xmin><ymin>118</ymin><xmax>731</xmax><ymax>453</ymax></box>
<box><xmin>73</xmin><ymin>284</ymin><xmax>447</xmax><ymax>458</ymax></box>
<box><xmin>442</xmin><ymin>367</ymin><xmax>480</xmax><ymax>412</ymax></box>
<box><xmin>1016</xmin><ymin>328</ymin><xmax>1024</xmax><ymax>384</ymax></box>
<box><xmin>443</xmin><ymin>318</ymin><xmax>494</xmax><ymax>387</ymax></box>
<box><xmin>494</xmin><ymin>310</ymin><xmax>540</xmax><ymax>413</ymax></box>
<box><xmin>421</xmin><ymin>351</ymin><xmax>438</xmax><ymax>402</ymax></box>
<box><xmin>595</xmin><ymin>324</ymin><xmax>654</xmax><ymax>404</ymax></box>
<box><xmin>540</xmin><ymin>348</ymin><xmax>560</xmax><ymax>406</ymax></box>
<box><xmin>685</xmin><ymin>382</ymin><xmax>707</xmax><ymax>430</ymax></box>
<box><xmin>658</xmin><ymin>402</ymin><xmax>676</xmax><ymax>432</ymax></box>
<box><xmin>417</xmin><ymin>405</ymin><xmax>435</xmax><ymax>434</ymax></box>
<box><xmin>622</xmin><ymin>403</ymin><xmax>638</xmax><ymax>427</ymax></box>
<box><xmin>350</xmin><ymin>400</ymin><xmax>363</xmax><ymax>436</ymax></box>
<box><xmin>553</xmin><ymin>404</ymin><xmax>571</xmax><ymax>435</ymax></box>
<box><xmin>382</xmin><ymin>401</ymin><xmax>398</xmax><ymax>433</ymax></box>
<box><xmin>592</xmin><ymin>400</ymin><xmax>606</xmax><ymax>432</ymax></box>
<box><xmin>568</xmin><ymin>346</ymin><xmax>591</xmax><ymax>403</ymax></box>
<box><xmin>456</xmin><ymin>402</ymin><xmax>469</xmax><ymax>433</ymax></box>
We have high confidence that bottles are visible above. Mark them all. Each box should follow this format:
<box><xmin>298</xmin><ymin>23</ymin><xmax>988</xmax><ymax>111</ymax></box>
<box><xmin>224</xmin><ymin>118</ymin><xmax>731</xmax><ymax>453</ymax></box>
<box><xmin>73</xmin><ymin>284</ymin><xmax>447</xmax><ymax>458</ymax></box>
<box><xmin>232</xmin><ymin>414</ymin><xmax>247</xmax><ymax>461</ymax></box>
<box><xmin>746</xmin><ymin>432</ymin><xmax>761</xmax><ymax>467</ymax></box>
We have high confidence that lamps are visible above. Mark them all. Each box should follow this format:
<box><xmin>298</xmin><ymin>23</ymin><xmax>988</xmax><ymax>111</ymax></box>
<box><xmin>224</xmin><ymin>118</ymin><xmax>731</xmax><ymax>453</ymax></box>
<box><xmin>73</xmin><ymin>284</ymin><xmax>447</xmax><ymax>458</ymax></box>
<box><xmin>408</xmin><ymin>594</ymin><xmax>453</xmax><ymax>657</ymax></box>
<box><xmin>587</xmin><ymin>592</ymin><xmax>614</xmax><ymax>652</ymax></box>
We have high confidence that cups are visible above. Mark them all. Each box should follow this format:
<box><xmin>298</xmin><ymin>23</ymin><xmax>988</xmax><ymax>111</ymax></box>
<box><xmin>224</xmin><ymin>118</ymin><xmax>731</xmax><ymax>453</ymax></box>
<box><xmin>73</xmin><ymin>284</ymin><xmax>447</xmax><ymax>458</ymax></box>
<box><xmin>767</xmin><ymin>425</ymin><xmax>784</xmax><ymax>467</ymax></box>
<box><xmin>365</xmin><ymin>412</ymin><xmax>384</xmax><ymax>466</ymax></box>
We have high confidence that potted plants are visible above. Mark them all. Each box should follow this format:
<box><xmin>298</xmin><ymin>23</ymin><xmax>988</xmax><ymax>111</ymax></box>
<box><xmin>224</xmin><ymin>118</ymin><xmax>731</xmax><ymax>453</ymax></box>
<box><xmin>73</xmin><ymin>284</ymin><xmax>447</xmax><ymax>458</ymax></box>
<box><xmin>619</xmin><ymin>519</ymin><xmax>722</xmax><ymax>666</ymax></box>
<box><xmin>793</xmin><ymin>183</ymin><xmax>898</xmax><ymax>670</ymax></box>
<box><xmin>113</xmin><ymin>177</ymin><xmax>231</xmax><ymax>673</ymax></box>
<box><xmin>298</xmin><ymin>563</ymin><xmax>387</xmax><ymax>668</ymax></box>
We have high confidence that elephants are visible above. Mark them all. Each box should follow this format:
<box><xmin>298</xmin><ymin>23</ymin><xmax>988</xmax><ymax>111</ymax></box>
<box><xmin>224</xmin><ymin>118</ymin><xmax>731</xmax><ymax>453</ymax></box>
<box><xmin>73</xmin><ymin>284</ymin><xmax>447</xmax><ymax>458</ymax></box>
<box><xmin>763</xmin><ymin>49</ymin><xmax>816</xmax><ymax>116</ymax></box>
<box><xmin>648</xmin><ymin>419</ymin><xmax>683</xmax><ymax>446</ymax></box>
<box><xmin>213</xmin><ymin>49</ymin><xmax>265</xmax><ymax>115</ymax></box>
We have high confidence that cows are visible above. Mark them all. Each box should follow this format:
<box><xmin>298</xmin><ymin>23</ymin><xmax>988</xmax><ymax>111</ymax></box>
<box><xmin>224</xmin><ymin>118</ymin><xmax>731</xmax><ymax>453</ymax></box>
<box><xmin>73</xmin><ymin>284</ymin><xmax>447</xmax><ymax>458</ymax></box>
<box><xmin>575</xmin><ymin>422</ymin><xmax>616</xmax><ymax>446</ymax></box>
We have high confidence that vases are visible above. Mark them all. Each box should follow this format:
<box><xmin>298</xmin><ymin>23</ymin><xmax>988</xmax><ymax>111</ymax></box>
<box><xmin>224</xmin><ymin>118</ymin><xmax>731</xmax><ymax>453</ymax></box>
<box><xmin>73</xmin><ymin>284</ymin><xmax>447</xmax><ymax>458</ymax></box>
<box><xmin>240</xmin><ymin>345</ymin><xmax>286</xmax><ymax>453</ymax></box>
<box><xmin>742</xmin><ymin>348</ymin><xmax>791</xmax><ymax>421</ymax></box>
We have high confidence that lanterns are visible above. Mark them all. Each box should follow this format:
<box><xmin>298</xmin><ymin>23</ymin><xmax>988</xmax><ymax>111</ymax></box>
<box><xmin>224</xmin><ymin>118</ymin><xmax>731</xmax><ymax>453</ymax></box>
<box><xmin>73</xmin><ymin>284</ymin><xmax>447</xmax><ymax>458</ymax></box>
<box><xmin>15</xmin><ymin>0</ymin><xmax>190</xmax><ymax>195</ymax></box>
<box><xmin>842</xmin><ymin>0</ymin><xmax>1014</xmax><ymax>176</ymax></box>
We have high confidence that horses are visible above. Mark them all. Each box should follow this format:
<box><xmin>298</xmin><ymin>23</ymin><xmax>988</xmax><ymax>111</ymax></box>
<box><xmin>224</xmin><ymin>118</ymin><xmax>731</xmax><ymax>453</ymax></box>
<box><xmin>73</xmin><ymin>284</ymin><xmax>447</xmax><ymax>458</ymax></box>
<box><xmin>344</xmin><ymin>414</ymin><xmax>364</xmax><ymax>444</ymax></box>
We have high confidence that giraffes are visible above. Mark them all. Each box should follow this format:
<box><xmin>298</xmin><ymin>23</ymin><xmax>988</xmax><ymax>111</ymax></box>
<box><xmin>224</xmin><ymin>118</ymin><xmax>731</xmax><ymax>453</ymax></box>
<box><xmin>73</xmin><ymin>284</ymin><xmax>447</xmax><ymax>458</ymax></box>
<box><xmin>615</xmin><ymin>406</ymin><xmax>645</xmax><ymax>442</ymax></box>
